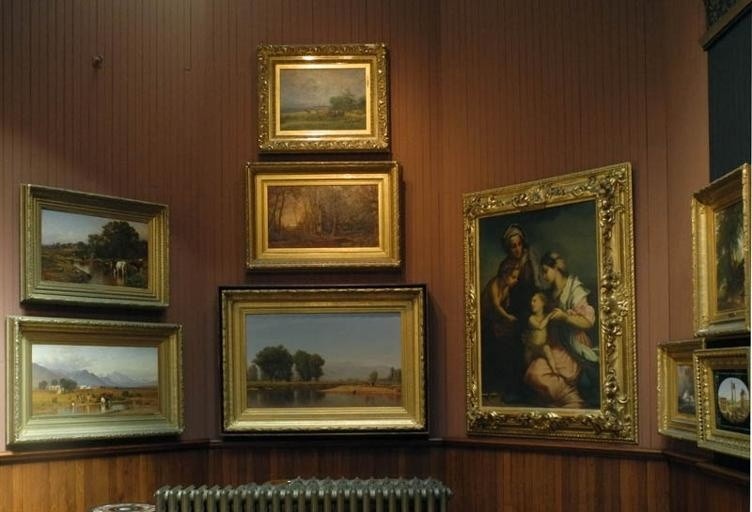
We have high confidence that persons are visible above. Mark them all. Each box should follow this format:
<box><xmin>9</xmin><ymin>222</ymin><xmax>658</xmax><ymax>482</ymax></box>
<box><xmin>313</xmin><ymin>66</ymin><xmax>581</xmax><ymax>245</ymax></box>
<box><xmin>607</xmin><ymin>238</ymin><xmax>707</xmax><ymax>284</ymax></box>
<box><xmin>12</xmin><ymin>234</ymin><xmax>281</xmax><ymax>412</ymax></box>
<box><xmin>479</xmin><ymin>223</ymin><xmax>596</xmax><ymax>409</ymax></box>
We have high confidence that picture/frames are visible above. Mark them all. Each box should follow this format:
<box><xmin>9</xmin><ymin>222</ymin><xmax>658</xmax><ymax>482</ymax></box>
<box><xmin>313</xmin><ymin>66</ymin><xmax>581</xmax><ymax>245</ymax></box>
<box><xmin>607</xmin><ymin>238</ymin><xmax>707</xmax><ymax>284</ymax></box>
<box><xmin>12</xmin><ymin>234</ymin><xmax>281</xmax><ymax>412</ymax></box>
<box><xmin>19</xmin><ymin>183</ymin><xmax>170</xmax><ymax>308</ymax></box>
<box><xmin>460</xmin><ymin>161</ymin><xmax>640</xmax><ymax>446</ymax></box>
<box><xmin>217</xmin><ymin>284</ymin><xmax>429</xmax><ymax>436</ymax></box>
<box><xmin>244</xmin><ymin>161</ymin><xmax>402</xmax><ymax>271</ymax></box>
<box><xmin>4</xmin><ymin>315</ymin><xmax>182</xmax><ymax>447</ymax></box>
<box><xmin>654</xmin><ymin>161</ymin><xmax>749</xmax><ymax>462</ymax></box>
<box><xmin>255</xmin><ymin>43</ymin><xmax>392</xmax><ymax>154</ymax></box>
